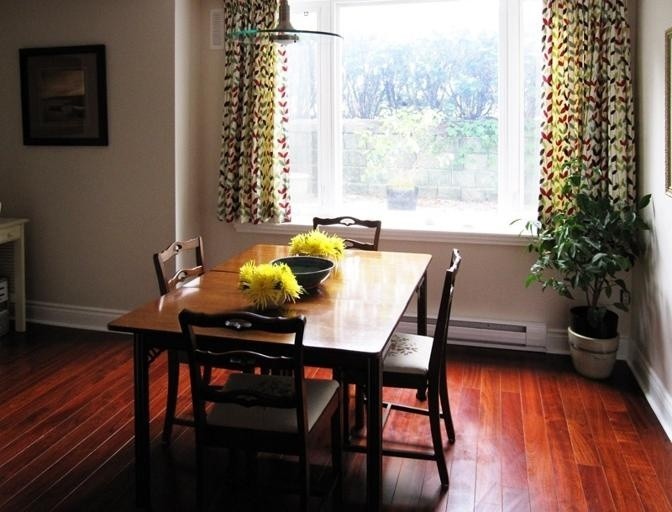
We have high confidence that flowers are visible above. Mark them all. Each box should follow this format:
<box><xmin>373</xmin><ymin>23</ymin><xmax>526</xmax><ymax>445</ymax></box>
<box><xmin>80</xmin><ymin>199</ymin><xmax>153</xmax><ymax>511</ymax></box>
<box><xmin>288</xmin><ymin>227</ymin><xmax>346</xmax><ymax>263</ymax></box>
<box><xmin>239</xmin><ymin>259</ymin><xmax>309</xmax><ymax>310</ymax></box>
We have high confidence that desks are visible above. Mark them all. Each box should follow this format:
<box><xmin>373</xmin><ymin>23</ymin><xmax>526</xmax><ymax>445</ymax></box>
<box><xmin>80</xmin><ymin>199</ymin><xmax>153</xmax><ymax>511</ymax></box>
<box><xmin>108</xmin><ymin>243</ymin><xmax>434</xmax><ymax>506</ymax></box>
<box><xmin>0</xmin><ymin>217</ymin><xmax>30</xmax><ymax>332</ymax></box>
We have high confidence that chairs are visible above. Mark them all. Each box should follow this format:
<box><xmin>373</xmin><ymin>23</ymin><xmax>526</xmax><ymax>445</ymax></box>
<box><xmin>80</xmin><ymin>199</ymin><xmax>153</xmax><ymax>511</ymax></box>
<box><xmin>177</xmin><ymin>308</ymin><xmax>344</xmax><ymax>509</ymax></box>
<box><xmin>154</xmin><ymin>235</ymin><xmax>274</xmax><ymax>440</ymax></box>
<box><xmin>330</xmin><ymin>248</ymin><xmax>462</xmax><ymax>488</ymax></box>
<box><xmin>269</xmin><ymin>216</ymin><xmax>382</xmax><ymax>415</ymax></box>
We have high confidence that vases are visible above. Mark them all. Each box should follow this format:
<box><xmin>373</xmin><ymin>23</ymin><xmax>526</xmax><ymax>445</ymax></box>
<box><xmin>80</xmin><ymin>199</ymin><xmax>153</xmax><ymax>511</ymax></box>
<box><xmin>249</xmin><ymin>288</ymin><xmax>287</xmax><ymax>309</ymax></box>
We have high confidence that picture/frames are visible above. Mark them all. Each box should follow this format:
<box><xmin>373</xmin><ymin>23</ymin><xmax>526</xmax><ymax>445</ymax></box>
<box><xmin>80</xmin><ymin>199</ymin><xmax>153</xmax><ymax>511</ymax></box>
<box><xmin>665</xmin><ymin>27</ymin><xmax>672</xmax><ymax>198</ymax></box>
<box><xmin>19</xmin><ymin>44</ymin><xmax>108</xmax><ymax>146</ymax></box>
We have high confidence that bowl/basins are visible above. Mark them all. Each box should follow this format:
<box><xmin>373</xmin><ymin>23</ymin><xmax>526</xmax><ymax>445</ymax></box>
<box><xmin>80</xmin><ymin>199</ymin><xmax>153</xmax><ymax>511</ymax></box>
<box><xmin>271</xmin><ymin>256</ymin><xmax>335</xmax><ymax>289</ymax></box>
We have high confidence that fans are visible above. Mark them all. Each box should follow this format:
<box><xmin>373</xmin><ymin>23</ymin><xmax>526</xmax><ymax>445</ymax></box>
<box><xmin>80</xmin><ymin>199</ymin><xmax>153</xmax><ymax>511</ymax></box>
<box><xmin>227</xmin><ymin>1</ymin><xmax>344</xmax><ymax>49</ymax></box>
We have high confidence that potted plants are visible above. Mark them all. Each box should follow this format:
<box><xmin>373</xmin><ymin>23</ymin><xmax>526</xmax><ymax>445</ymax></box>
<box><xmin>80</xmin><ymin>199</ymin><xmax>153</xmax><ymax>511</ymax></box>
<box><xmin>510</xmin><ymin>158</ymin><xmax>651</xmax><ymax>380</ymax></box>
